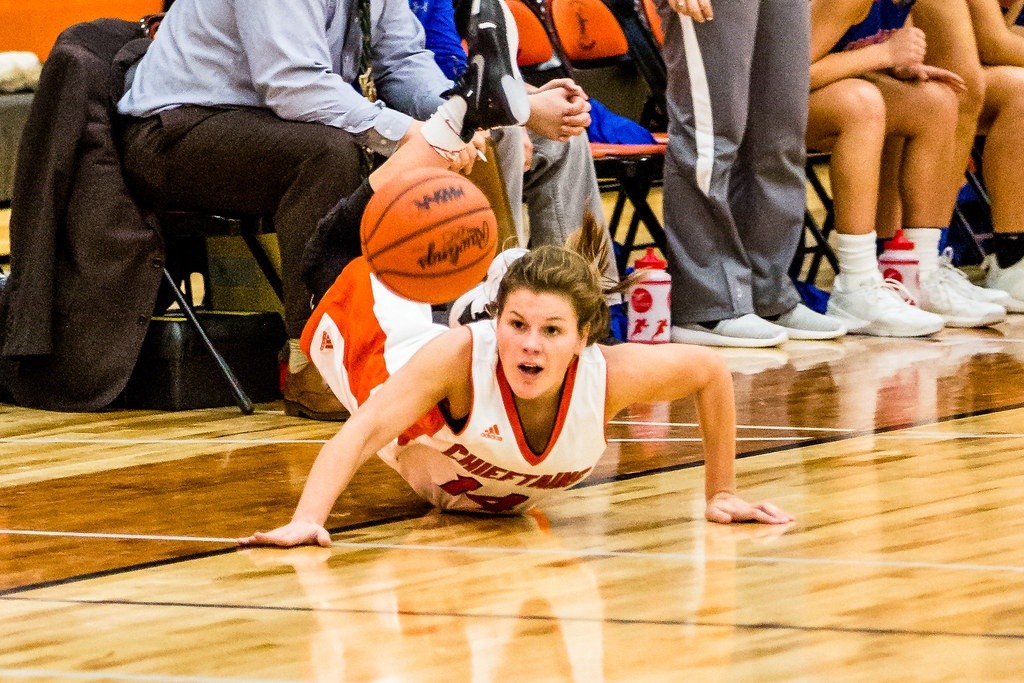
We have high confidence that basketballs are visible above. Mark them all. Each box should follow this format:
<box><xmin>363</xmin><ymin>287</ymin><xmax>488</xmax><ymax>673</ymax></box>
<box><xmin>357</xmin><ymin>162</ymin><xmax>501</xmax><ymax>308</ymax></box>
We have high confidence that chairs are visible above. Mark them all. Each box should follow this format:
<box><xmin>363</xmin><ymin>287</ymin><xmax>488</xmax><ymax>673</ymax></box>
<box><xmin>460</xmin><ymin>0</ymin><xmax>669</xmax><ymax>276</ymax></box>
<box><xmin>545</xmin><ymin>0</ymin><xmax>671</xmax><ymax>271</ymax></box>
<box><xmin>63</xmin><ymin>19</ymin><xmax>285</xmax><ymax>414</ymax></box>
<box><xmin>632</xmin><ymin>0</ymin><xmax>668</xmax><ymax>53</ymax></box>
<box><xmin>801</xmin><ymin>150</ymin><xmax>843</xmax><ymax>287</ymax></box>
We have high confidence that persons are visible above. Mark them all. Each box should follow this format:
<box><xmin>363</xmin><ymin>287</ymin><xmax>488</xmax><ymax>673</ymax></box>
<box><xmin>650</xmin><ymin>1</ymin><xmax>850</xmax><ymax>350</ymax></box>
<box><xmin>240</xmin><ymin>1</ymin><xmax>794</xmax><ymax>549</ymax></box>
<box><xmin>120</xmin><ymin>1</ymin><xmax>538</xmax><ymax>422</ymax></box>
<box><xmin>808</xmin><ymin>1</ymin><xmax>1007</xmax><ymax>342</ymax></box>
<box><xmin>879</xmin><ymin>0</ymin><xmax>1024</xmax><ymax>315</ymax></box>
<box><xmin>398</xmin><ymin>0</ymin><xmax>623</xmax><ymax>312</ymax></box>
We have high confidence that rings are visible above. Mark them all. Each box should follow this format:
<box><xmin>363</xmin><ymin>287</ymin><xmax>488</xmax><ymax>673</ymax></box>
<box><xmin>677</xmin><ymin>2</ymin><xmax>685</xmax><ymax>7</ymax></box>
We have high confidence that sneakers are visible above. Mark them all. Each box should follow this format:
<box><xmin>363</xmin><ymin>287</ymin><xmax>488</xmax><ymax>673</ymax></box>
<box><xmin>768</xmin><ymin>304</ymin><xmax>846</xmax><ymax>339</ymax></box>
<box><xmin>667</xmin><ymin>313</ymin><xmax>787</xmax><ymax>350</ymax></box>
<box><xmin>916</xmin><ymin>271</ymin><xmax>1007</xmax><ymax>328</ymax></box>
<box><xmin>928</xmin><ymin>258</ymin><xmax>1011</xmax><ymax>308</ymax></box>
<box><xmin>983</xmin><ymin>253</ymin><xmax>1024</xmax><ymax>315</ymax></box>
<box><xmin>823</xmin><ymin>278</ymin><xmax>944</xmax><ymax>339</ymax></box>
<box><xmin>446</xmin><ymin>0</ymin><xmax>529</xmax><ymax>135</ymax></box>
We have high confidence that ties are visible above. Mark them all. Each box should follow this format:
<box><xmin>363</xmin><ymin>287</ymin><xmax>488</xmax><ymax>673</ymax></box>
<box><xmin>340</xmin><ymin>1</ymin><xmax>368</xmax><ymax>87</ymax></box>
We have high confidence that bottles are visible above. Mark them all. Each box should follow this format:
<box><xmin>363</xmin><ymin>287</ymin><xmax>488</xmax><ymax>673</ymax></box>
<box><xmin>624</xmin><ymin>248</ymin><xmax>673</xmax><ymax>344</ymax></box>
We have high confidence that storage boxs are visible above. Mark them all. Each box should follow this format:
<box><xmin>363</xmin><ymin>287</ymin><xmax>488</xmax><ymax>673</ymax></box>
<box><xmin>140</xmin><ymin>307</ymin><xmax>283</xmax><ymax>412</ymax></box>
